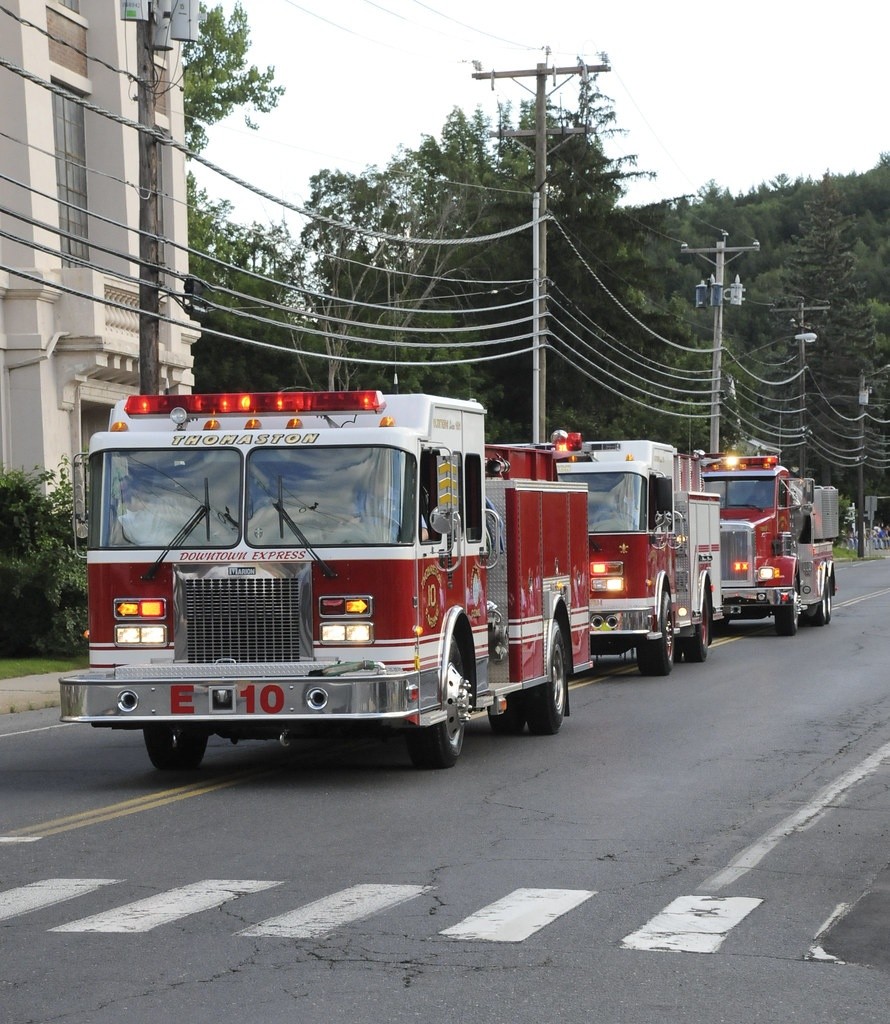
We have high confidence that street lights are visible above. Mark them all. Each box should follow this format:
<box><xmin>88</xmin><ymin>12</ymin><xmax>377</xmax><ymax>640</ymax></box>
<box><xmin>709</xmin><ymin>332</ymin><xmax>817</xmax><ymax>454</ymax></box>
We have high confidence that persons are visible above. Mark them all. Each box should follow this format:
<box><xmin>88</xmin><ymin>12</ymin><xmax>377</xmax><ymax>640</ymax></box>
<box><xmin>848</xmin><ymin>520</ymin><xmax>890</xmax><ymax>550</ymax></box>
<box><xmin>338</xmin><ymin>464</ymin><xmax>428</xmax><ymax>542</ymax></box>
<box><xmin>594</xmin><ymin>486</ymin><xmax>640</xmax><ymax>529</ymax></box>
<box><xmin>745</xmin><ymin>484</ymin><xmax>772</xmax><ymax>506</ymax></box>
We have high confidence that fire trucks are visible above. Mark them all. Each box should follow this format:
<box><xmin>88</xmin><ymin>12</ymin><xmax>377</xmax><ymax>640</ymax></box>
<box><xmin>58</xmin><ymin>388</ymin><xmax>596</xmax><ymax>769</ymax></box>
<box><xmin>490</xmin><ymin>429</ymin><xmax>727</xmax><ymax>677</ymax></box>
<box><xmin>691</xmin><ymin>449</ymin><xmax>840</xmax><ymax>636</ymax></box>
